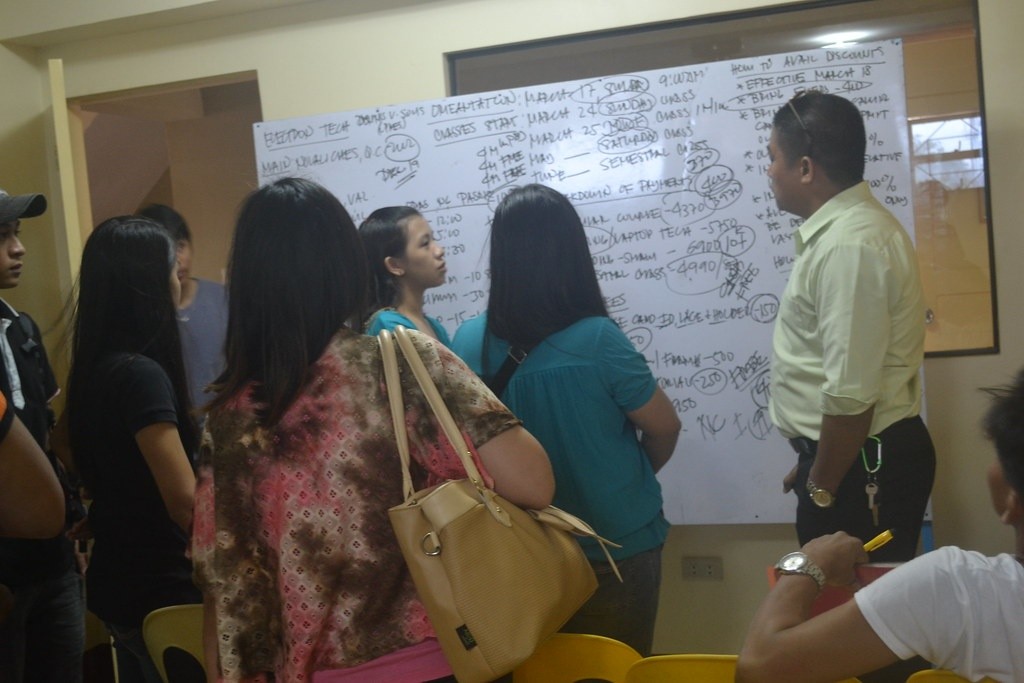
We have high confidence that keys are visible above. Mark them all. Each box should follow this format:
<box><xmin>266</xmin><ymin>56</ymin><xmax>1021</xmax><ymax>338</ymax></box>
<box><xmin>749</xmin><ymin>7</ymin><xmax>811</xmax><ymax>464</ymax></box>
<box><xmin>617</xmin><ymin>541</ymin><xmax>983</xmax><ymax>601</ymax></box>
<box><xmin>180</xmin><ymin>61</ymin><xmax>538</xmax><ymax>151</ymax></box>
<box><xmin>863</xmin><ymin>476</ymin><xmax>885</xmax><ymax>527</ymax></box>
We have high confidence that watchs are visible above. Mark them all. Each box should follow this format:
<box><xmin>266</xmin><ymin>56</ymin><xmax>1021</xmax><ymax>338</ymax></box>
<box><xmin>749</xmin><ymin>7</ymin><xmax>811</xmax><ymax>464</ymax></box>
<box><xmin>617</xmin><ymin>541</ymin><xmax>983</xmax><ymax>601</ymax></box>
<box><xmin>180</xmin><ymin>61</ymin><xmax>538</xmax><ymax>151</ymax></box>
<box><xmin>771</xmin><ymin>551</ymin><xmax>826</xmax><ymax>589</ymax></box>
<box><xmin>805</xmin><ymin>477</ymin><xmax>836</xmax><ymax>509</ymax></box>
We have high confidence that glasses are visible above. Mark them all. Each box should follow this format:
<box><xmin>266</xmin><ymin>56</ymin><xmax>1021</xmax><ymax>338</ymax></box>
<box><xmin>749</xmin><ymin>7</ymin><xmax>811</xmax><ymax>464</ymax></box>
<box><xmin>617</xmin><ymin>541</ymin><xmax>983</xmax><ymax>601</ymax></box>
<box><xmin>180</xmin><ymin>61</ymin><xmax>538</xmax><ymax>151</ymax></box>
<box><xmin>790</xmin><ymin>90</ymin><xmax>816</xmax><ymax>153</ymax></box>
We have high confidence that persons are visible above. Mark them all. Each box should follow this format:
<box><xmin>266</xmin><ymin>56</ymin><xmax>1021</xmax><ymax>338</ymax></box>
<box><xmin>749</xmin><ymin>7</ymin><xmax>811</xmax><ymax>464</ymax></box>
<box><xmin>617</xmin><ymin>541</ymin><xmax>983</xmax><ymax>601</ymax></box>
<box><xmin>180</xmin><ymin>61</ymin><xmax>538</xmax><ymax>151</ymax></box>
<box><xmin>0</xmin><ymin>181</ymin><xmax>86</xmax><ymax>683</ymax></box>
<box><xmin>133</xmin><ymin>201</ymin><xmax>245</xmax><ymax>414</ymax></box>
<box><xmin>765</xmin><ymin>89</ymin><xmax>939</xmax><ymax>566</ymax></box>
<box><xmin>54</xmin><ymin>214</ymin><xmax>202</xmax><ymax>683</ymax></box>
<box><xmin>734</xmin><ymin>367</ymin><xmax>1024</xmax><ymax>683</ymax></box>
<box><xmin>448</xmin><ymin>182</ymin><xmax>684</xmax><ymax>659</ymax></box>
<box><xmin>183</xmin><ymin>175</ymin><xmax>557</xmax><ymax>683</ymax></box>
<box><xmin>356</xmin><ymin>205</ymin><xmax>456</xmax><ymax>349</ymax></box>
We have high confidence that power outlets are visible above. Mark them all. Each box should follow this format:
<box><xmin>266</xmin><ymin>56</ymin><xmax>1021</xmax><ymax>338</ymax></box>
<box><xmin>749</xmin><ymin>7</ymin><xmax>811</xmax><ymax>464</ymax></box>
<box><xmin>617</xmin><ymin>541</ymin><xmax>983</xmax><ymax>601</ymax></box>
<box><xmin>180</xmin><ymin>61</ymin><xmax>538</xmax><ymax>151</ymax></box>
<box><xmin>680</xmin><ymin>554</ymin><xmax>723</xmax><ymax>582</ymax></box>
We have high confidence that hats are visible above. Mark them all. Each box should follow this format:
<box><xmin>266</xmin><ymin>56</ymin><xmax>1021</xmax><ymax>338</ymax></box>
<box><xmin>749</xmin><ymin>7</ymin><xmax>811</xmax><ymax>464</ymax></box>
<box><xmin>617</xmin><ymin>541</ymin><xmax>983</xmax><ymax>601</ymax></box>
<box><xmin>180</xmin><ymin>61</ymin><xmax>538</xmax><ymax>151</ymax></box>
<box><xmin>0</xmin><ymin>191</ymin><xmax>48</xmax><ymax>222</ymax></box>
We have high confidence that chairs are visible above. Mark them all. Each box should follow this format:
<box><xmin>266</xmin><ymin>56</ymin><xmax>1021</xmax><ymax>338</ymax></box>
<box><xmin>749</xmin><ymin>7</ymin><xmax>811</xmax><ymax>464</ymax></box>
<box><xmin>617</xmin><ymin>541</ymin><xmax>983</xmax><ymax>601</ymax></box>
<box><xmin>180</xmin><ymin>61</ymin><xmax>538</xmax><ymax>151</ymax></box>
<box><xmin>513</xmin><ymin>633</ymin><xmax>1003</xmax><ymax>682</ymax></box>
<box><xmin>142</xmin><ymin>604</ymin><xmax>210</xmax><ymax>683</ymax></box>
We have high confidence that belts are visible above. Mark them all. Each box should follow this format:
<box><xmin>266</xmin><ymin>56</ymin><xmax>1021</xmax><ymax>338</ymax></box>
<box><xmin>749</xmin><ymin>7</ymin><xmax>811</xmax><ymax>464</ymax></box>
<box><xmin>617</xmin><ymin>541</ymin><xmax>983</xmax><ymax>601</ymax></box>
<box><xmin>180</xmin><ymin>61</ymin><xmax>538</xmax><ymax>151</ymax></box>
<box><xmin>789</xmin><ymin>437</ymin><xmax>819</xmax><ymax>454</ymax></box>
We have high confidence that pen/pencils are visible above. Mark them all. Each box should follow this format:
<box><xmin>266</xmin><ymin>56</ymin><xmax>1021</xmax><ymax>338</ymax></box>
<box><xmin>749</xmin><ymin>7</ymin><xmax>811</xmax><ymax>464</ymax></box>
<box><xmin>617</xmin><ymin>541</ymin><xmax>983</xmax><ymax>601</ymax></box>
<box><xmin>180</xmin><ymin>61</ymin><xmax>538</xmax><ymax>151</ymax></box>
<box><xmin>863</xmin><ymin>528</ymin><xmax>897</xmax><ymax>552</ymax></box>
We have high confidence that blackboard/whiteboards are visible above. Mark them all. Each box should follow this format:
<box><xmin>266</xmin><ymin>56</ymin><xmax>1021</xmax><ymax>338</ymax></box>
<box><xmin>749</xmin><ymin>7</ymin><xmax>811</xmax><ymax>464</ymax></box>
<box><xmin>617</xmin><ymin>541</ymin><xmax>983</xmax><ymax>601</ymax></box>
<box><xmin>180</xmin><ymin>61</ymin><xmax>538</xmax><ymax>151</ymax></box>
<box><xmin>251</xmin><ymin>38</ymin><xmax>933</xmax><ymax>526</ymax></box>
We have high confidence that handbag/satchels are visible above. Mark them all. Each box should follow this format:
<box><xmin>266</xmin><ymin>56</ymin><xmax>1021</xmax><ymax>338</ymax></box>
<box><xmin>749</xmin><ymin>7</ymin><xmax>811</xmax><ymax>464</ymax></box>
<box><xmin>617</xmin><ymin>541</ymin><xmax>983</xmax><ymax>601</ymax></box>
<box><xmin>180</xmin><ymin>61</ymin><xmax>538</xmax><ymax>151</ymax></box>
<box><xmin>376</xmin><ymin>324</ymin><xmax>625</xmax><ymax>683</ymax></box>
